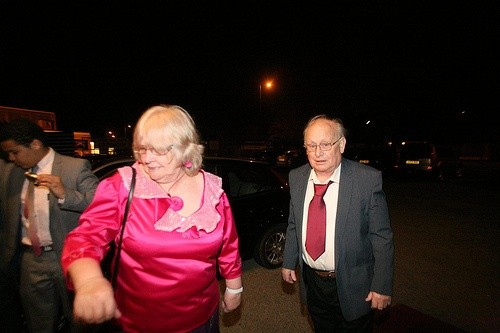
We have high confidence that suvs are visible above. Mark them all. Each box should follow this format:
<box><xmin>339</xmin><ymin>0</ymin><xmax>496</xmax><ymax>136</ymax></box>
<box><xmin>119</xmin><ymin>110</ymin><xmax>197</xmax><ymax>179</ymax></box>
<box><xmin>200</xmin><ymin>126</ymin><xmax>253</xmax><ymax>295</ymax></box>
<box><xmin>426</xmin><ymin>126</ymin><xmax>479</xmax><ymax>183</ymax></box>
<box><xmin>395</xmin><ymin>141</ymin><xmax>441</xmax><ymax>178</ymax></box>
<box><xmin>354</xmin><ymin>150</ymin><xmax>387</xmax><ymax>176</ymax></box>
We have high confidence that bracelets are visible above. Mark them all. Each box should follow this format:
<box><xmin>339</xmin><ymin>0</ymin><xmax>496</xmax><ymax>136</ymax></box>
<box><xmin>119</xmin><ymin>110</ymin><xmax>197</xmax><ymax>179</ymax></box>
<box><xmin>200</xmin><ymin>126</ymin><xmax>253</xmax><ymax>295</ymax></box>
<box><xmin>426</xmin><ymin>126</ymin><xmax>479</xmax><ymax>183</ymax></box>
<box><xmin>226</xmin><ymin>287</ymin><xmax>243</xmax><ymax>294</ymax></box>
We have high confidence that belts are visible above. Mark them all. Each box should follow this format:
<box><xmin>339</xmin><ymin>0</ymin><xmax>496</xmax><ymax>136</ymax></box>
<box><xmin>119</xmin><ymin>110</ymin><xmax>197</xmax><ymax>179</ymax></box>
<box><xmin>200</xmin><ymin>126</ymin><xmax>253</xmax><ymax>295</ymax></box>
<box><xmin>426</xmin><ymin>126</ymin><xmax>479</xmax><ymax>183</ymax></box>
<box><xmin>314</xmin><ymin>270</ymin><xmax>336</xmax><ymax>277</ymax></box>
<box><xmin>20</xmin><ymin>243</ymin><xmax>54</xmax><ymax>252</ymax></box>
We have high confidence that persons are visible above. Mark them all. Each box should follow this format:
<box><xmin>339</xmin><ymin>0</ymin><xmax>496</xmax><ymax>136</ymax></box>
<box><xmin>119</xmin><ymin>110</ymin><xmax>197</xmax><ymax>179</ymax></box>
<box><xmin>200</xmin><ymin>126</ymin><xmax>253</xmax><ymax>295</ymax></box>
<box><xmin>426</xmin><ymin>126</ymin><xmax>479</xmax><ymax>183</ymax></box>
<box><xmin>61</xmin><ymin>106</ymin><xmax>243</xmax><ymax>333</ymax></box>
<box><xmin>0</xmin><ymin>116</ymin><xmax>100</xmax><ymax>333</ymax></box>
<box><xmin>281</xmin><ymin>114</ymin><xmax>394</xmax><ymax>333</ymax></box>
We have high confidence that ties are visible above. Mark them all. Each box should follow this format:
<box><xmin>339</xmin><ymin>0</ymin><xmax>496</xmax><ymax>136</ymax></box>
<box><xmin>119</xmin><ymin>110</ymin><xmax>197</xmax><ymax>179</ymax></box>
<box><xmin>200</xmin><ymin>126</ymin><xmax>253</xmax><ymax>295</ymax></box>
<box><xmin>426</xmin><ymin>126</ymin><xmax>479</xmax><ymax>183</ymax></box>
<box><xmin>306</xmin><ymin>180</ymin><xmax>333</xmax><ymax>262</ymax></box>
<box><xmin>24</xmin><ymin>164</ymin><xmax>42</xmax><ymax>256</ymax></box>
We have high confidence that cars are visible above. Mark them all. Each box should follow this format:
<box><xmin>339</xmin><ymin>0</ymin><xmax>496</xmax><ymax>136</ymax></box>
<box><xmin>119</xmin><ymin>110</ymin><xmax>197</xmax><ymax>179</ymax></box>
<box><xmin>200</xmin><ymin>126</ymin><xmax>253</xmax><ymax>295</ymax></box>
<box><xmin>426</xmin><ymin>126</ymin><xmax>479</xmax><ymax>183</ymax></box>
<box><xmin>90</xmin><ymin>156</ymin><xmax>292</xmax><ymax>270</ymax></box>
<box><xmin>273</xmin><ymin>143</ymin><xmax>307</xmax><ymax>172</ymax></box>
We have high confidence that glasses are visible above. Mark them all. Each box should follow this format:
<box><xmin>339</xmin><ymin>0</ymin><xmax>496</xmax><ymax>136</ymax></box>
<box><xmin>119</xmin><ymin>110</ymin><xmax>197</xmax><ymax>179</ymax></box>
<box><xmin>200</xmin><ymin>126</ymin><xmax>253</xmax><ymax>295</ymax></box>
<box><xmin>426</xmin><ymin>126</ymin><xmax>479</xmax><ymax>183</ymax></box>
<box><xmin>136</xmin><ymin>145</ymin><xmax>172</xmax><ymax>155</ymax></box>
<box><xmin>304</xmin><ymin>136</ymin><xmax>344</xmax><ymax>151</ymax></box>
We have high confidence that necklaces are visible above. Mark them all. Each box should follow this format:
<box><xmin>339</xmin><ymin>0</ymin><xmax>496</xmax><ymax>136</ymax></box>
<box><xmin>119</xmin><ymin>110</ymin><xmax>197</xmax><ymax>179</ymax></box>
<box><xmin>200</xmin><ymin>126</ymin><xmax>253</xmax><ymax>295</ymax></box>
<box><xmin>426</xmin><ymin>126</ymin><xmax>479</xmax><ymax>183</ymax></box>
<box><xmin>167</xmin><ymin>174</ymin><xmax>184</xmax><ymax>197</ymax></box>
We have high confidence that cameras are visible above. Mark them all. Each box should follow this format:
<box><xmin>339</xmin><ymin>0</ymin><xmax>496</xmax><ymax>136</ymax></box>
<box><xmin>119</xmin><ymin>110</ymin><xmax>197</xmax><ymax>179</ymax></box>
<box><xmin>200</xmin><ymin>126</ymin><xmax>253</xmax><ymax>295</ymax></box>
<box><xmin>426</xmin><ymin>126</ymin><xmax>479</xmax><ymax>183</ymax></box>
<box><xmin>25</xmin><ymin>172</ymin><xmax>41</xmax><ymax>186</ymax></box>
